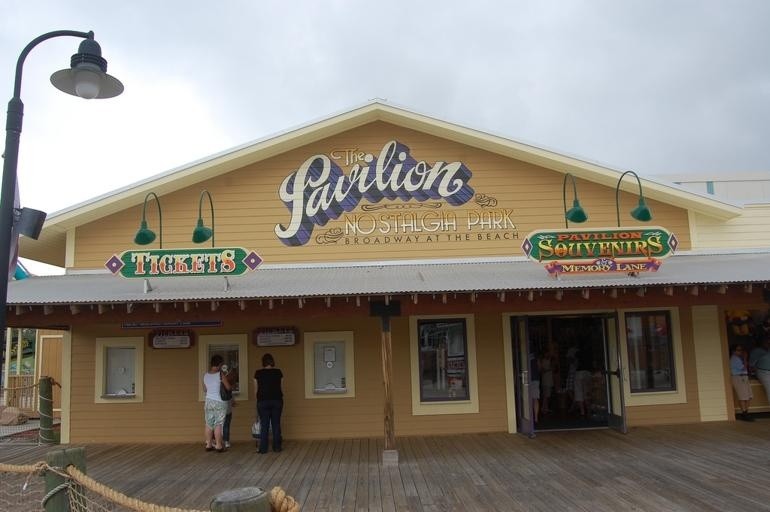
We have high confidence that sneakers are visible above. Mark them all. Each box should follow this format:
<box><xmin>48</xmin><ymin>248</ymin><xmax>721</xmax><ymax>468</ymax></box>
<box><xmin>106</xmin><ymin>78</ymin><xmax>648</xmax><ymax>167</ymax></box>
<box><xmin>205</xmin><ymin>439</ymin><xmax>230</xmax><ymax>451</ymax></box>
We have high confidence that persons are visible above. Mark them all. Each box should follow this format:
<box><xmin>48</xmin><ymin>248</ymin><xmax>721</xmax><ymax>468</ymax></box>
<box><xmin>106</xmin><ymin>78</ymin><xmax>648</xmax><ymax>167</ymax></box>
<box><xmin>729</xmin><ymin>344</ymin><xmax>756</xmax><ymax>422</ymax></box>
<box><xmin>201</xmin><ymin>354</ymin><xmax>232</xmax><ymax>453</ymax></box>
<box><xmin>530</xmin><ymin>337</ymin><xmax>606</xmax><ymax>427</ymax></box>
<box><xmin>210</xmin><ymin>364</ymin><xmax>232</xmax><ymax>449</ymax></box>
<box><xmin>252</xmin><ymin>353</ymin><xmax>285</xmax><ymax>454</ymax></box>
<box><xmin>748</xmin><ymin>335</ymin><xmax>770</xmax><ymax>406</ymax></box>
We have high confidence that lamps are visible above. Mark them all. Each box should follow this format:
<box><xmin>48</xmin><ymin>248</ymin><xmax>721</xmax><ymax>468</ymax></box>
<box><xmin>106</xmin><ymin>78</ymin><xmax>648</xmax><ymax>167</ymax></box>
<box><xmin>563</xmin><ymin>172</ymin><xmax>587</xmax><ymax>229</ymax></box>
<box><xmin>192</xmin><ymin>190</ymin><xmax>214</xmax><ymax>247</ymax></box>
<box><xmin>134</xmin><ymin>192</ymin><xmax>162</xmax><ymax>249</ymax></box>
<box><xmin>615</xmin><ymin>171</ymin><xmax>652</xmax><ymax>227</ymax></box>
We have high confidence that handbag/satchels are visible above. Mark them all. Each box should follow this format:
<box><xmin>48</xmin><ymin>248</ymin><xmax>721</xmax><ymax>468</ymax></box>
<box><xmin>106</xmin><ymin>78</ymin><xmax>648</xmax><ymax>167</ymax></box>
<box><xmin>251</xmin><ymin>417</ymin><xmax>273</xmax><ymax>438</ymax></box>
<box><xmin>220</xmin><ymin>381</ymin><xmax>233</xmax><ymax>402</ymax></box>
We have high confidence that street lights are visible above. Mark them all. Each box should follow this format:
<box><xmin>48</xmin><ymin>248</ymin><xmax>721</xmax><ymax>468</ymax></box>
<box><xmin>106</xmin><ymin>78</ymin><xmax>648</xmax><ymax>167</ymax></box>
<box><xmin>2</xmin><ymin>29</ymin><xmax>127</xmax><ymax>397</ymax></box>
